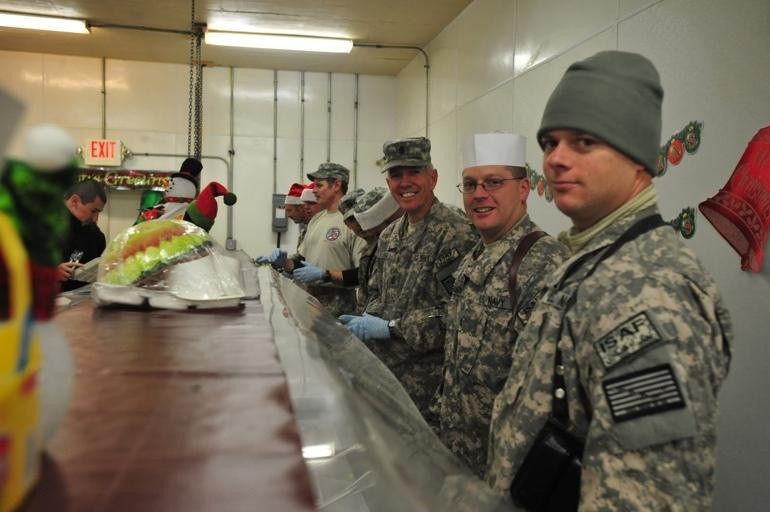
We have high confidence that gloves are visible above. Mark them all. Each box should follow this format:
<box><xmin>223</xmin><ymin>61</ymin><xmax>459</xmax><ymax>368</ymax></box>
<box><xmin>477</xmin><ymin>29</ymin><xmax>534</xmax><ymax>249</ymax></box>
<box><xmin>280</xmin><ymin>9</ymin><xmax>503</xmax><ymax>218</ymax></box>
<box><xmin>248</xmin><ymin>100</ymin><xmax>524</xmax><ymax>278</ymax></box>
<box><xmin>255</xmin><ymin>255</ymin><xmax>274</xmax><ymax>266</ymax></box>
<box><xmin>338</xmin><ymin>310</ymin><xmax>392</xmax><ymax>345</ymax></box>
<box><xmin>293</xmin><ymin>261</ymin><xmax>322</xmax><ymax>284</ymax></box>
<box><xmin>267</xmin><ymin>248</ymin><xmax>288</xmax><ymax>266</ymax></box>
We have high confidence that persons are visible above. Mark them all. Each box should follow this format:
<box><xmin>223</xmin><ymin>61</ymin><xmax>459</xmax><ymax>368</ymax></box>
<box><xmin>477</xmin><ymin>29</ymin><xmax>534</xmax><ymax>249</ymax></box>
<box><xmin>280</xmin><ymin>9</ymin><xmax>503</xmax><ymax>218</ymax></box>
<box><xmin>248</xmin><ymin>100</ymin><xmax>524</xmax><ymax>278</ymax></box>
<box><xmin>485</xmin><ymin>50</ymin><xmax>733</xmax><ymax>512</ymax></box>
<box><xmin>0</xmin><ymin>122</ymin><xmax>106</xmax><ymax>323</ymax></box>
<box><xmin>163</xmin><ymin>159</ymin><xmax>203</xmax><ymax>197</ymax></box>
<box><xmin>339</xmin><ymin>136</ymin><xmax>480</xmax><ymax>421</ymax></box>
<box><xmin>431</xmin><ymin>133</ymin><xmax>569</xmax><ymax>482</ymax></box>
<box><xmin>256</xmin><ymin>162</ymin><xmax>402</xmax><ymax>315</ymax></box>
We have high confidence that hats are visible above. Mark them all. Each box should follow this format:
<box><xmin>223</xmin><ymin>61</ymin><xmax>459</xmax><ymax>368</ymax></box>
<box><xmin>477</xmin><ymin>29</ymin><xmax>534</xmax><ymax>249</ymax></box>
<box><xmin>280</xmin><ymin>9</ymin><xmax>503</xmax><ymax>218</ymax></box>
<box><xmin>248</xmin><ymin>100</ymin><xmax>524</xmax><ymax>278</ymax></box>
<box><xmin>356</xmin><ymin>185</ymin><xmax>398</xmax><ymax>231</ymax></box>
<box><xmin>459</xmin><ymin>131</ymin><xmax>526</xmax><ymax>169</ymax></box>
<box><xmin>337</xmin><ymin>187</ymin><xmax>365</xmax><ymax>222</ymax></box>
<box><xmin>285</xmin><ymin>182</ymin><xmax>305</xmax><ymax>206</ymax></box>
<box><xmin>186</xmin><ymin>181</ymin><xmax>237</xmax><ymax>232</ymax></box>
<box><xmin>379</xmin><ymin>136</ymin><xmax>432</xmax><ymax>173</ymax></box>
<box><xmin>538</xmin><ymin>50</ymin><xmax>665</xmax><ymax>177</ymax></box>
<box><xmin>300</xmin><ymin>183</ymin><xmax>318</xmax><ymax>203</ymax></box>
<box><xmin>306</xmin><ymin>162</ymin><xmax>349</xmax><ymax>184</ymax></box>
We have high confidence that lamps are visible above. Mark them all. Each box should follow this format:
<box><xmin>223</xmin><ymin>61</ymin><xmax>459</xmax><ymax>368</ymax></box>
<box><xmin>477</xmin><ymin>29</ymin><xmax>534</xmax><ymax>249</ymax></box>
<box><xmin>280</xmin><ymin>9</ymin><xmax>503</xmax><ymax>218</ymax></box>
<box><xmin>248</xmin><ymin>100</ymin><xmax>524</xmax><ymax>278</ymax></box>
<box><xmin>203</xmin><ymin>31</ymin><xmax>355</xmax><ymax>54</ymax></box>
<box><xmin>0</xmin><ymin>12</ymin><xmax>92</xmax><ymax>34</ymax></box>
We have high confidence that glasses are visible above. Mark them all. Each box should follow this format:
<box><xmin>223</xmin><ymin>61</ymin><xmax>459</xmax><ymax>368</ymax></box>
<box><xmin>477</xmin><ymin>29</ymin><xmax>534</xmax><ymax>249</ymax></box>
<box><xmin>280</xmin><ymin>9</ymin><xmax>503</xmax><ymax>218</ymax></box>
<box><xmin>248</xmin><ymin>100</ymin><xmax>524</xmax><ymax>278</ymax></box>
<box><xmin>456</xmin><ymin>175</ymin><xmax>524</xmax><ymax>194</ymax></box>
